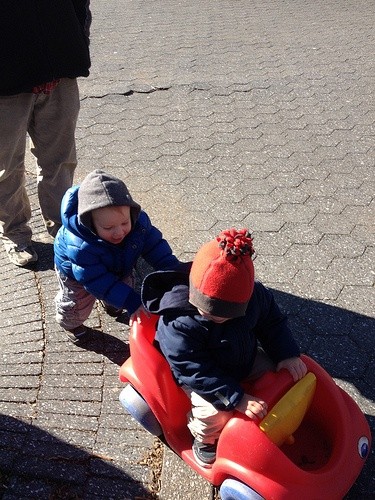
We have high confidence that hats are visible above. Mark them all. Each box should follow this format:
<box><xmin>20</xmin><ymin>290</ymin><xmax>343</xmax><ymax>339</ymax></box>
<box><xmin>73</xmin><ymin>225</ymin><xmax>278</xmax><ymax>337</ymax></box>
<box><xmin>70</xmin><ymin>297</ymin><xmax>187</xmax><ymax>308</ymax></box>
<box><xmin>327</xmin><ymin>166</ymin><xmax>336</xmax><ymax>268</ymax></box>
<box><xmin>188</xmin><ymin>227</ymin><xmax>257</xmax><ymax>318</ymax></box>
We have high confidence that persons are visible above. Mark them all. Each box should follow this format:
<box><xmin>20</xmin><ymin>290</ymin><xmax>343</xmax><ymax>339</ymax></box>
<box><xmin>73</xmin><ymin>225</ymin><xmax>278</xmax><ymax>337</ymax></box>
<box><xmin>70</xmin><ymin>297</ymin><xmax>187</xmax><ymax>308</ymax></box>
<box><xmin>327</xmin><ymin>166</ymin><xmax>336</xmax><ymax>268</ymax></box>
<box><xmin>0</xmin><ymin>0</ymin><xmax>92</xmax><ymax>268</ymax></box>
<box><xmin>54</xmin><ymin>170</ymin><xmax>185</xmax><ymax>346</ymax></box>
<box><xmin>140</xmin><ymin>228</ymin><xmax>307</xmax><ymax>469</ymax></box>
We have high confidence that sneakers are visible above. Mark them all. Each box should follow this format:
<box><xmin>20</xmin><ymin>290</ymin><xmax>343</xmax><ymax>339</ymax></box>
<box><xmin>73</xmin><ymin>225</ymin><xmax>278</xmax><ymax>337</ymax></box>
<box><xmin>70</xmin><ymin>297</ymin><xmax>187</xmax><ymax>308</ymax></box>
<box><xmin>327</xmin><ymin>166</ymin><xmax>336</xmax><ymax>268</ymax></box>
<box><xmin>2</xmin><ymin>239</ymin><xmax>39</xmax><ymax>270</ymax></box>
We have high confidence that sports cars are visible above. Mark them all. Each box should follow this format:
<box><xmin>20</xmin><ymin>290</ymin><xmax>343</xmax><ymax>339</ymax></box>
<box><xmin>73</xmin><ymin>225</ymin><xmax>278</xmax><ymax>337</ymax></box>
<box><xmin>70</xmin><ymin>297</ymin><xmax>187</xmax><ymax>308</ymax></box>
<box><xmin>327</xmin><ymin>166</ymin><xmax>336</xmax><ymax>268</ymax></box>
<box><xmin>117</xmin><ymin>299</ymin><xmax>374</xmax><ymax>500</ymax></box>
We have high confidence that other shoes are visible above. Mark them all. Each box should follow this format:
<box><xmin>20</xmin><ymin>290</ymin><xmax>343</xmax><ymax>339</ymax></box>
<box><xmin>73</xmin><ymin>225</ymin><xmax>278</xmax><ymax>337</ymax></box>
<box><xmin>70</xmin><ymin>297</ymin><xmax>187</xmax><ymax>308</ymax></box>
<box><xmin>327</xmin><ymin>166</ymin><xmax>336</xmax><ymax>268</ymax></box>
<box><xmin>64</xmin><ymin>322</ymin><xmax>92</xmax><ymax>346</ymax></box>
<box><xmin>192</xmin><ymin>439</ymin><xmax>216</xmax><ymax>469</ymax></box>
<box><xmin>100</xmin><ymin>299</ymin><xmax>123</xmax><ymax>317</ymax></box>
